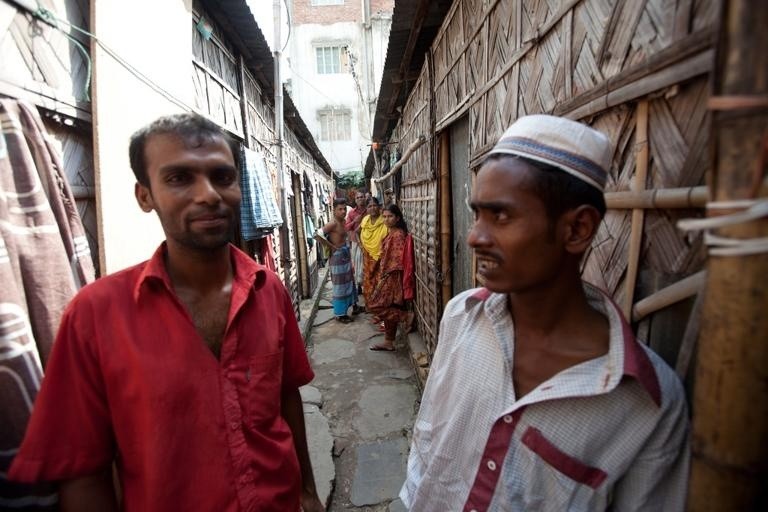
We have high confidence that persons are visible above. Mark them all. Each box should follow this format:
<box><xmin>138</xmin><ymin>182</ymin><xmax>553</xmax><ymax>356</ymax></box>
<box><xmin>313</xmin><ymin>189</ymin><xmax>417</xmax><ymax>352</ymax></box>
<box><xmin>6</xmin><ymin>113</ymin><xmax>333</xmax><ymax>511</ymax></box>
<box><xmin>397</xmin><ymin>114</ymin><xmax>691</xmax><ymax>511</ymax></box>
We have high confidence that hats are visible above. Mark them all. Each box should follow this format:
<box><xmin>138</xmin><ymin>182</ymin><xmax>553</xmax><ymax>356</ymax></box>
<box><xmin>486</xmin><ymin>114</ymin><xmax>612</xmax><ymax>194</ymax></box>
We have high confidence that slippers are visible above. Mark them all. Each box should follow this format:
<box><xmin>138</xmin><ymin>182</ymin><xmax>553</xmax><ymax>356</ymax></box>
<box><xmin>352</xmin><ymin>305</ymin><xmax>366</xmax><ymax>315</ymax></box>
<box><xmin>379</xmin><ymin>320</ymin><xmax>386</xmax><ymax>332</ymax></box>
<box><xmin>336</xmin><ymin>315</ymin><xmax>354</xmax><ymax>324</ymax></box>
<box><xmin>372</xmin><ymin>315</ymin><xmax>381</xmax><ymax>324</ymax></box>
<box><xmin>369</xmin><ymin>343</ymin><xmax>395</xmax><ymax>351</ymax></box>
